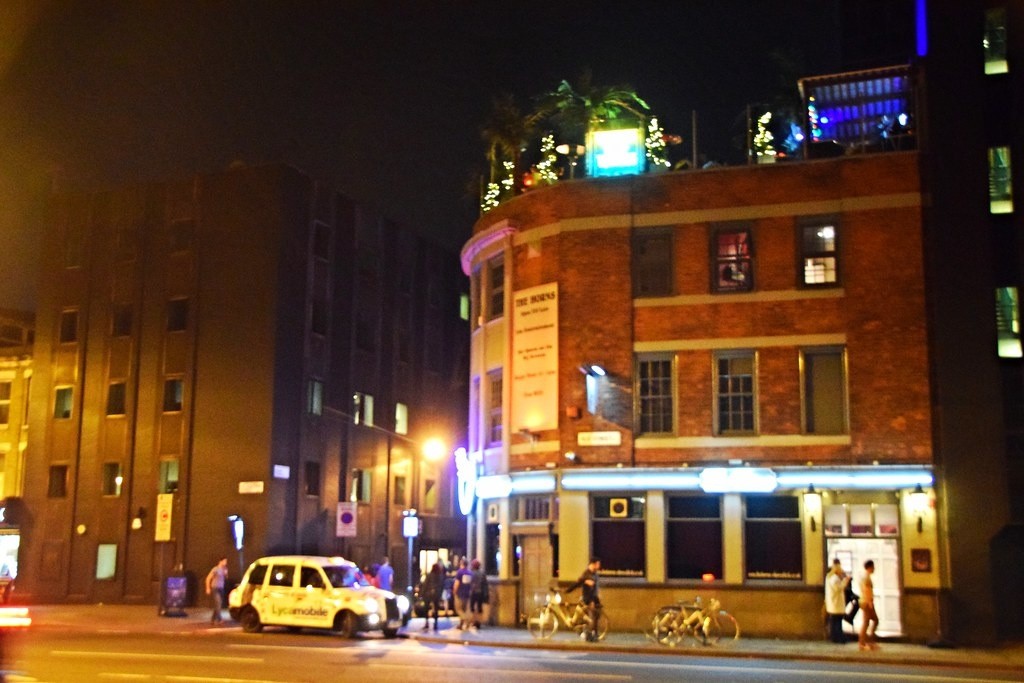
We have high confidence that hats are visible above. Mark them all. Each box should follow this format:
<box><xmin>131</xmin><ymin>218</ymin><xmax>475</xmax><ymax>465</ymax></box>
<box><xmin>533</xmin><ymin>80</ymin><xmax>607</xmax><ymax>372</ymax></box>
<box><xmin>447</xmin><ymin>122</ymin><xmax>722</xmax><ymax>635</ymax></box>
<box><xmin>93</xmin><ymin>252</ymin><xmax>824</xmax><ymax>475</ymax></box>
<box><xmin>472</xmin><ymin>559</ymin><xmax>480</xmax><ymax>569</ymax></box>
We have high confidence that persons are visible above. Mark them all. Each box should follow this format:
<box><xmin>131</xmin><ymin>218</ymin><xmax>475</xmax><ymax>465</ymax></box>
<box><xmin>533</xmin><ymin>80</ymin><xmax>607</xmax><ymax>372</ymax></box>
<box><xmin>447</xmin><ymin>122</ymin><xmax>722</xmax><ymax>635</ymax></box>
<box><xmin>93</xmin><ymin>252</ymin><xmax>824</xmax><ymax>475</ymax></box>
<box><xmin>467</xmin><ymin>560</ymin><xmax>487</xmax><ymax>627</ymax></box>
<box><xmin>440</xmin><ymin>561</ymin><xmax>456</xmax><ymax>617</ymax></box>
<box><xmin>363</xmin><ymin>556</ymin><xmax>394</xmax><ymax>592</ymax></box>
<box><xmin>857</xmin><ymin>559</ymin><xmax>879</xmax><ymax>651</ymax></box>
<box><xmin>874</xmin><ymin>114</ymin><xmax>890</xmax><ymax>150</ymax></box>
<box><xmin>207</xmin><ymin>556</ymin><xmax>230</xmax><ymax>625</ymax></box>
<box><xmin>824</xmin><ymin>559</ymin><xmax>854</xmax><ymax>644</ymax></box>
<box><xmin>422</xmin><ymin>563</ymin><xmax>444</xmax><ymax>631</ymax></box>
<box><xmin>567</xmin><ymin>559</ymin><xmax>602</xmax><ymax>642</ymax></box>
<box><xmin>452</xmin><ymin>559</ymin><xmax>475</xmax><ymax>630</ymax></box>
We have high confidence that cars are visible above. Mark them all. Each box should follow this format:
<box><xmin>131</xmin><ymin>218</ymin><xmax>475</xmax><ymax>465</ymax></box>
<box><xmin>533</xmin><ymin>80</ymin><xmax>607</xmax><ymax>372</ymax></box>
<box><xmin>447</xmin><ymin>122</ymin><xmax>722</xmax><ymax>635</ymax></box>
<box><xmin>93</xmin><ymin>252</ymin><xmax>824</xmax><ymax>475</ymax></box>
<box><xmin>228</xmin><ymin>587</ymin><xmax>243</xmax><ymax>625</ymax></box>
<box><xmin>237</xmin><ymin>554</ymin><xmax>412</xmax><ymax>636</ymax></box>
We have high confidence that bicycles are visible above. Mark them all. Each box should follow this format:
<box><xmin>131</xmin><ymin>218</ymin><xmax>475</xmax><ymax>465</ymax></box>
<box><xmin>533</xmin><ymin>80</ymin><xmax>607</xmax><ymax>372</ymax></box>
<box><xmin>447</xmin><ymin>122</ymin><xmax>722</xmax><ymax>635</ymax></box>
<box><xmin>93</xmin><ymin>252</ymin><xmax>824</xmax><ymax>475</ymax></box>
<box><xmin>651</xmin><ymin>597</ymin><xmax>742</xmax><ymax>648</ymax></box>
<box><xmin>526</xmin><ymin>585</ymin><xmax>609</xmax><ymax>643</ymax></box>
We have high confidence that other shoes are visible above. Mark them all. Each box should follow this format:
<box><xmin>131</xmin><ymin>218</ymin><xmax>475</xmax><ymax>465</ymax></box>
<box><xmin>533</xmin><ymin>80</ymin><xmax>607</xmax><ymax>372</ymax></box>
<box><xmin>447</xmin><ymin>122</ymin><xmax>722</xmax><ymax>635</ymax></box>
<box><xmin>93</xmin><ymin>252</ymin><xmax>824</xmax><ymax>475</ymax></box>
<box><xmin>424</xmin><ymin>621</ymin><xmax>481</xmax><ymax>630</ymax></box>
